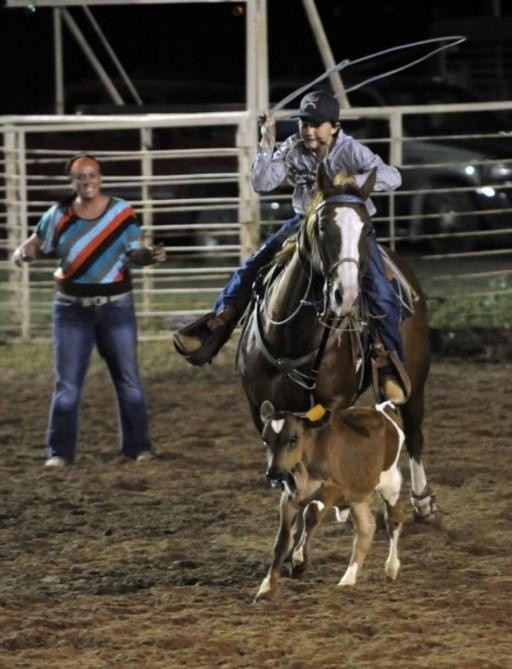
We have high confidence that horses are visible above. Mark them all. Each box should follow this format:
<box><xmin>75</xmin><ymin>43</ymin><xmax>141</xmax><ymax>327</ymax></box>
<box><xmin>237</xmin><ymin>162</ymin><xmax>440</xmax><ymax>525</ymax></box>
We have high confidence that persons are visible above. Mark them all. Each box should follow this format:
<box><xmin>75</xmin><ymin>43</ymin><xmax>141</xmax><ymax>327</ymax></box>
<box><xmin>8</xmin><ymin>150</ymin><xmax>168</xmax><ymax>467</ymax></box>
<box><xmin>171</xmin><ymin>89</ymin><xmax>415</xmax><ymax>405</ymax></box>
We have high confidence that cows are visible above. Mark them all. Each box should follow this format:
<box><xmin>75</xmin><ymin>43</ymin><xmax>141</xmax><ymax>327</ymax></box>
<box><xmin>252</xmin><ymin>400</ymin><xmax>413</xmax><ymax>607</ymax></box>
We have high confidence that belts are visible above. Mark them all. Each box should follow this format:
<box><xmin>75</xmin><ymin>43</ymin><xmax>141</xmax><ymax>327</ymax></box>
<box><xmin>57</xmin><ymin>293</ymin><xmax>129</xmax><ymax>307</ymax></box>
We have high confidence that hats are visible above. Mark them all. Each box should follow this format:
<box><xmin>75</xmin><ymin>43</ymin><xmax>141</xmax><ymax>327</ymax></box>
<box><xmin>291</xmin><ymin>91</ymin><xmax>340</xmax><ymax>123</ymax></box>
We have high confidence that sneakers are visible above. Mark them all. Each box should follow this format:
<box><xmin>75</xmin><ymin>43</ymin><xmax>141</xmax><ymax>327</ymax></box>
<box><xmin>171</xmin><ymin>331</ymin><xmax>204</xmax><ymax>356</ymax></box>
<box><xmin>45</xmin><ymin>455</ymin><xmax>68</xmax><ymax>468</ymax></box>
<box><xmin>135</xmin><ymin>450</ymin><xmax>156</xmax><ymax>464</ymax></box>
<box><xmin>378</xmin><ymin>375</ymin><xmax>407</xmax><ymax>404</ymax></box>
